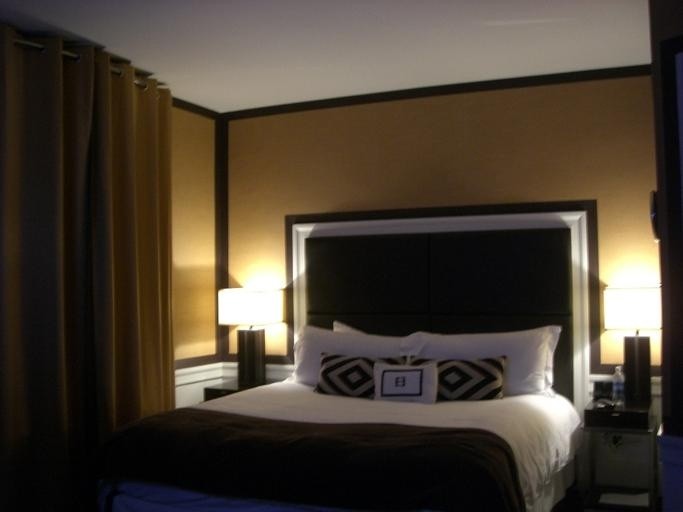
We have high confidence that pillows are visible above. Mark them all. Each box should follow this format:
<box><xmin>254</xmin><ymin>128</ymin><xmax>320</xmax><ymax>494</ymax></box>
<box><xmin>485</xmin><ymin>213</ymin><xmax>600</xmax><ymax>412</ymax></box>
<box><xmin>290</xmin><ymin>317</ymin><xmax>563</xmax><ymax>403</ymax></box>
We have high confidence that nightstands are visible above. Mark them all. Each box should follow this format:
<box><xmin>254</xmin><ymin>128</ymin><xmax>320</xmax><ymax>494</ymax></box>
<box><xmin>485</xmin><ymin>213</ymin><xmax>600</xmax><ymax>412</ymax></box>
<box><xmin>581</xmin><ymin>397</ymin><xmax>659</xmax><ymax>511</ymax></box>
<box><xmin>204</xmin><ymin>378</ymin><xmax>284</xmax><ymax>401</ymax></box>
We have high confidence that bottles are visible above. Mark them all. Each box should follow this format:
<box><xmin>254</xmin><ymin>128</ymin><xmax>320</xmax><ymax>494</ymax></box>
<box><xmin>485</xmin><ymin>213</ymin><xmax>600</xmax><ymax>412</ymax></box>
<box><xmin>612</xmin><ymin>365</ymin><xmax>625</xmax><ymax>409</ymax></box>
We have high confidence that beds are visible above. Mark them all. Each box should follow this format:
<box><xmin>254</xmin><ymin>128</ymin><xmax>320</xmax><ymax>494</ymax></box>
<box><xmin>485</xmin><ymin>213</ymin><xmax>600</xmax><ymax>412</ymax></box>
<box><xmin>114</xmin><ymin>198</ymin><xmax>601</xmax><ymax>512</ymax></box>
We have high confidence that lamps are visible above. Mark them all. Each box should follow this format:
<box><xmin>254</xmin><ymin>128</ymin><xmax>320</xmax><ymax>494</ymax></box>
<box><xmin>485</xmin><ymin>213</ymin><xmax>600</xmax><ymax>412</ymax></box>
<box><xmin>217</xmin><ymin>288</ymin><xmax>284</xmax><ymax>387</ymax></box>
